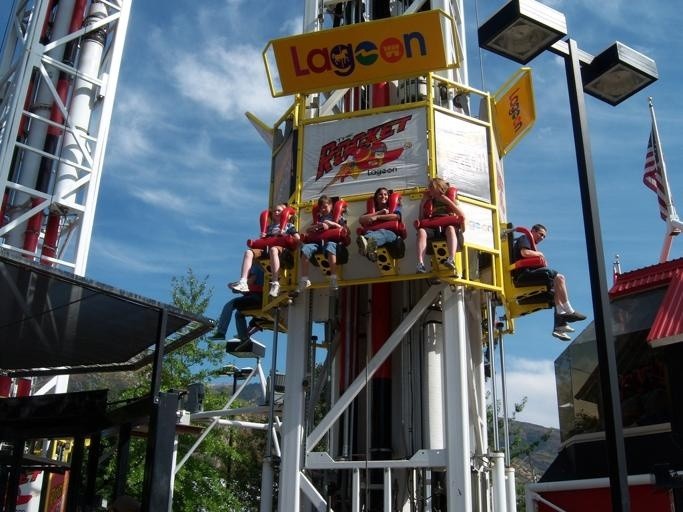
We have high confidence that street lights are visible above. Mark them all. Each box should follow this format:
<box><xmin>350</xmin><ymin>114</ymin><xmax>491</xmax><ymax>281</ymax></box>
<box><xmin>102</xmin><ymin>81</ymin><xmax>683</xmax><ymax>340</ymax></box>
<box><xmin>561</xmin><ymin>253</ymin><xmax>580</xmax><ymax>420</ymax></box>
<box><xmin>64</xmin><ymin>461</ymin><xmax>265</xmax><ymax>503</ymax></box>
<box><xmin>217</xmin><ymin>364</ymin><xmax>256</xmax><ymax>501</ymax></box>
<box><xmin>223</xmin><ymin>337</ymin><xmax>280</xmax><ymax>463</ymax></box>
<box><xmin>477</xmin><ymin>0</ymin><xmax>658</xmax><ymax>511</ymax></box>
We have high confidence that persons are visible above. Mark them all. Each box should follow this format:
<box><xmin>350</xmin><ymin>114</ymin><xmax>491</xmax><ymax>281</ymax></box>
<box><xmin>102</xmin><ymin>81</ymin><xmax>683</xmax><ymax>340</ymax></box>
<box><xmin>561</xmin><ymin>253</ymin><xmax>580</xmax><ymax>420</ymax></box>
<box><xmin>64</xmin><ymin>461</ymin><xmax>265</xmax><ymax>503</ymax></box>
<box><xmin>511</xmin><ymin>224</ymin><xmax>586</xmax><ymax>323</ymax></box>
<box><xmin>295</xmin><ymin>196</ymin><xmax>350</xmax><ymax>291</ymax></box>
<box><xmin>517</xmin><ymin>289</ymin><xmax>574</xmax><ymax>341</ymax></box>
<box><xmin>227</xmin><ymin>203</ymin><xmax>302</xmax><ymax>298</ymax></box>
<box><xmin>355</xmin><ymin>188</ymin><xmax>406</xmax><ymax>256</ymax></box>
<box><xmin>412</xmin><ymin>178</ymin><xmax>464</xmax><ymax>274</ymax></box>
<box><xmin>207</xmin><ymin>252</ymin><xmax>264</xmax><ymax>340</ymax></box>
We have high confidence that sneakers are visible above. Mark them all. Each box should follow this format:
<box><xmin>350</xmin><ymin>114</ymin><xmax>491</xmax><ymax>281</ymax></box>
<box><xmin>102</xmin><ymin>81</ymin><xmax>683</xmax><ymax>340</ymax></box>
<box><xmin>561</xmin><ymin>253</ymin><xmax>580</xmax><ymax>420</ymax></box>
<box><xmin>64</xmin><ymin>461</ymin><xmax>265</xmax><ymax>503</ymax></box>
<box><xmin>366</xmin><ymin>237</ymin><xmax>377</xmax><ymax>261</ymax></box>
<box><xmin>553</xmin><ymin>311</ymin><xmax>586</xmax><ymax>341</ymax></box>
<box><xmin>356</xmin><ymin>235</ymin><xmax>367</xmax><ymax>256</ymax></box>
<box><xmin>296</xmin><ymin>279</ymin><xmax>311</xmax><ymax>292</ymax></box>
<box><xmin>331</xmin><ymin>279</ymin><xmax>339</xmax><ymax>291</ymax></box>
<box><xmin>208</xmin><ymin>332</ymin><xmax>225</xmax><ymax>340</ymax></box>
<box><xmin>416</xmin><ymin>263</ymin><xmax>426</xmax><ymax>273</ymax></box>
<box><xmin>228</xmin><ymin>281</ymin><xmax>250</xmax><ymax>293</ymax></box>
<box><xmin>269</xmin><ymin>284</ymin><xmax>279</xmax><ymax>297</ymax></box>
<box><xmin>444</xmin><ymin>260</ymin><xmax>455</xmax><ymax>269</ymax></box>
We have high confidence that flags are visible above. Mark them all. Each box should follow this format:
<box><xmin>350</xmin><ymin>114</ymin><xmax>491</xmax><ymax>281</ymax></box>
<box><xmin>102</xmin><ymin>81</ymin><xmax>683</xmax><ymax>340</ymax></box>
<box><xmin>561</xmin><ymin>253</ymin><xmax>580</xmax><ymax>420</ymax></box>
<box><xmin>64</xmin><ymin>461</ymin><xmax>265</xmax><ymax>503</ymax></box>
<box><xmin>643</xmin><ymin>125</ymin><xmax>675</xmax><ymax>222</ymax></box>
